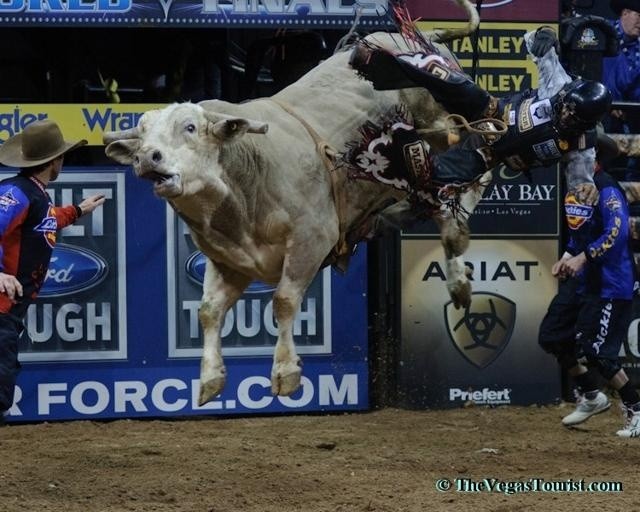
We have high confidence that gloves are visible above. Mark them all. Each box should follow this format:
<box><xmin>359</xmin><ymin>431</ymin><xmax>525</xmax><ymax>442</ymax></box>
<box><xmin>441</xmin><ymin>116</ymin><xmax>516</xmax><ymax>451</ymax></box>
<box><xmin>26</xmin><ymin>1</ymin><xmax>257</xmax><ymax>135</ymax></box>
<box><xmin>531</xmin><ymin>30</ymin><xmax>561</xmax><ymax>57</ymax></box>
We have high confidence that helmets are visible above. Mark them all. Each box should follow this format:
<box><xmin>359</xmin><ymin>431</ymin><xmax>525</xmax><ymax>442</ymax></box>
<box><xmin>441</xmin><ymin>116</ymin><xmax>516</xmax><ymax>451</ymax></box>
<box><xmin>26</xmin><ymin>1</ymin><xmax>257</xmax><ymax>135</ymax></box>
<box><xmin>563</xmin><ymin>80</ymin><xmax>610</xmax><ymax>124</ymax></box>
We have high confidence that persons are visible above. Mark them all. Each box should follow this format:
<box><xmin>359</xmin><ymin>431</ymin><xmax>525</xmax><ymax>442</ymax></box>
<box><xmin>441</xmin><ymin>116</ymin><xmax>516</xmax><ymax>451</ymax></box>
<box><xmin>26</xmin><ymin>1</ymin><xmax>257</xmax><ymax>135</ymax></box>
<box><xmin>422</xmin><ymin>22</ymin><xmax>613</xmax><ymax>215</ymax></box>
<box><xmin>536</xmin><ymin>153</ymin><xmax>640</xmax><ymax>443</ymax></box>
<box><xmin>0</xmin><ymin>119</ymin><xmax>107</xmax><ymax>406</ymax></box>
<box><xmin>587</xmin><ymin>2</ymin><xmax>639</xmax><ymax>137</ymax></box>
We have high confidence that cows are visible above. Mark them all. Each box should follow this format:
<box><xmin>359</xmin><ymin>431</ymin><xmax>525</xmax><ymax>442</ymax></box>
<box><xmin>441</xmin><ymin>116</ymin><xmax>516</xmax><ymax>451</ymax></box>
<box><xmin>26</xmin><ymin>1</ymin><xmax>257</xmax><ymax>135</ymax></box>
<box><xmin>101</xmin><ymin>0</ymin><xmax>493</xmax><ymax>408</ymax></box>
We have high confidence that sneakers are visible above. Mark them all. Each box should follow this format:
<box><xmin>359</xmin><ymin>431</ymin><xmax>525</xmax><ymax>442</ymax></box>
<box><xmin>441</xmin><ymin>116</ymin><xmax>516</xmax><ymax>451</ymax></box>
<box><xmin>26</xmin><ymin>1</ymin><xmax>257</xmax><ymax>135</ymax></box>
<box><xmin>562</xmin><ymin>391</ymin><xmax>611</xmax><ymax>425</ymax></box>
<box><xmin>349</xmin><ymin>44</ymin><xmax>371</xmax><ymax>65</ymax></box>
<box><xmin>616</xmin><ymin>408</ymin><xmax>640</xmax><ymax>437</ymax></box>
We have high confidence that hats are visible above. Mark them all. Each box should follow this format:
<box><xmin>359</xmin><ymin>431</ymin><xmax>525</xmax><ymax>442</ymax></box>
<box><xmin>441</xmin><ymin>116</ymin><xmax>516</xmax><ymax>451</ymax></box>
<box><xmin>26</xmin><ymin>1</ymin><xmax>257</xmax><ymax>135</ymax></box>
<box><xmin>609</xmin><ymin>1</ymin><xmax>640</xmax><ymax>15</ymax></box>
<box><xmin>1</xmin><ymin>119</ymin><xmax>88</xmax><ymax>168</ymax></box>
<box><xmin>595</xmin><ymin>134</ymin><xmax>617</xmax><ymax>167</ymax></box>
